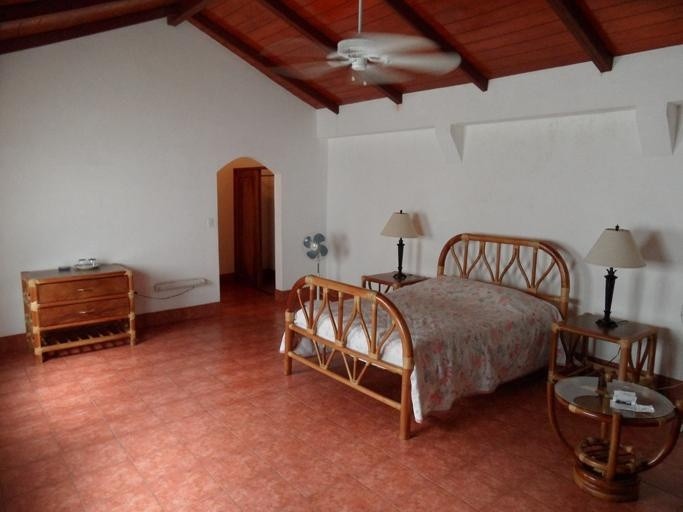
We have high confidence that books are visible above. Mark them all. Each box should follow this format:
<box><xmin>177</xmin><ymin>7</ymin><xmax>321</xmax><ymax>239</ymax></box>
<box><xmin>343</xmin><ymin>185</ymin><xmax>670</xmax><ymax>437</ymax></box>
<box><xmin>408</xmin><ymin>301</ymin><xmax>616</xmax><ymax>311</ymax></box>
<box><xmin>610</xmin><ymin>389</ymin><xmax>655</xmax><ymax>414</ymax></box>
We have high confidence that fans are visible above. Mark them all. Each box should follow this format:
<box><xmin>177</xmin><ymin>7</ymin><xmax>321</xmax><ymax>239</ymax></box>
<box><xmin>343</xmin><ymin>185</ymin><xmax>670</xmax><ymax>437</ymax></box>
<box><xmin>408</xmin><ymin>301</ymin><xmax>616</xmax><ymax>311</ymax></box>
<box><xmin>258</xmin><ymin>0</ymin><xmax>462</xmax><ymax>87</ymax></box>
<box><xmin>302</xmin><ymin>232</ymin><xmax>332</xmax><ymax>307</ymax></box>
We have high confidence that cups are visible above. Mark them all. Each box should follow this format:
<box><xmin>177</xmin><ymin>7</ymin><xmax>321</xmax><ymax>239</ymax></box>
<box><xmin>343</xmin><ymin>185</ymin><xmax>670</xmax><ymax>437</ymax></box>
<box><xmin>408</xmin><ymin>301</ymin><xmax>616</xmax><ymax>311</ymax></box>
<box><xmin>78</xmin><ymin>258</ymin><xmax>95</xmax><ymax>266</ymax></box>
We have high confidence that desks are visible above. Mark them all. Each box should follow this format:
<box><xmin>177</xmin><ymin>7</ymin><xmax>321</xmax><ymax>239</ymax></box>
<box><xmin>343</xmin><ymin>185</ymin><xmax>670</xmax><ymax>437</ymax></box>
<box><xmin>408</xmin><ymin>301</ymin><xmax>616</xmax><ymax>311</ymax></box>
<box><xmin>547</xmin><ymin>312</ymin><xmax>658</xmax><ymax>385</ymax></box>
<box><xmin>546</xmin><ymin>370</ymin><xmax>683</xmax><ymax>503</ymax></box>
<box><xmin>361</xmin><ymin>271</ymin><xmax>431</xmax><ymax>294</ymax></box>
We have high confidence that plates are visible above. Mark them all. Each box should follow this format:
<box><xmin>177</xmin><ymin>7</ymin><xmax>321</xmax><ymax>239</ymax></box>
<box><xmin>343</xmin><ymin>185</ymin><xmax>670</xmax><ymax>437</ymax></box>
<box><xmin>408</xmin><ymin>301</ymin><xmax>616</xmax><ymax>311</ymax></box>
<box><xmin>73</xmin><ymin>263</ymin><xmax>99</xmax><ymax>269</ymax></box>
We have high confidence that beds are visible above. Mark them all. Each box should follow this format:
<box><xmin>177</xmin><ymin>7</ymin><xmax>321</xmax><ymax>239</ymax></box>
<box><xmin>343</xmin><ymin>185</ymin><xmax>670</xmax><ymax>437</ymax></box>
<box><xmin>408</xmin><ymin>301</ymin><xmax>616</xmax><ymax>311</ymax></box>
<box><xmin>279</xmin><ymin>233</ymin><xmax>570</xmax><ymax>441</ymax></box>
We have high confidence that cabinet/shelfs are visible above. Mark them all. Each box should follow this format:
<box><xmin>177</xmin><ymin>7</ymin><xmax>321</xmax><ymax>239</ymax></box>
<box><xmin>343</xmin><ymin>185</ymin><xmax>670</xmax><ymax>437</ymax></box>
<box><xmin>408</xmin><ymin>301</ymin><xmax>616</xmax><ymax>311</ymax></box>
<box><xmin>21</xmin><ymin>264</ymin><xmax>136</xmax><ymax>364</ymax></box>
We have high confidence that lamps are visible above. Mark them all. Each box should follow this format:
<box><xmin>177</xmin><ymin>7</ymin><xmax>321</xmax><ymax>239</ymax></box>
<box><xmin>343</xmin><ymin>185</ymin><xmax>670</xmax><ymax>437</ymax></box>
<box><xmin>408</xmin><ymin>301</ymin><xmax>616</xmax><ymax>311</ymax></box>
<box><xmin>583</xmin><ymin>224</ymin><xmax>647</xmax><ymax>329</ymax></box>
<box><xmin>381</xmin><ymin>209</ymin><xmax>419</xmax><ymax>281</ymax></box>
